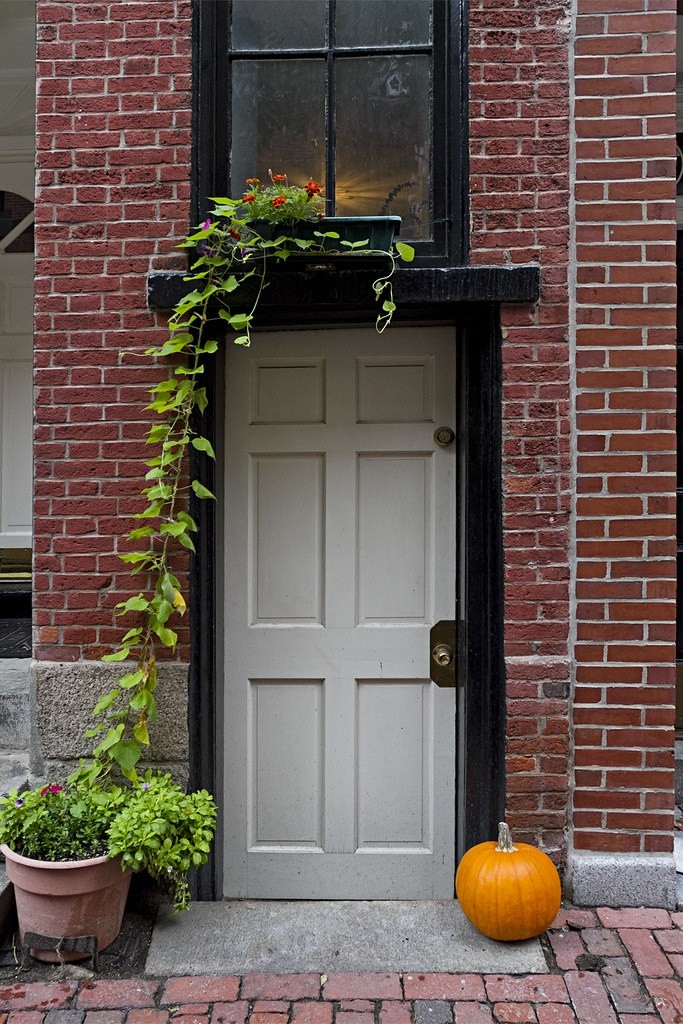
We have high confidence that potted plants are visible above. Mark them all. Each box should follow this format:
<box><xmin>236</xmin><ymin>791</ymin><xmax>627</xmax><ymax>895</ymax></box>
<box><xmin>0</xmin><ymin>777</ymin><xmax>216</xmax><ymax>961</ymax></box>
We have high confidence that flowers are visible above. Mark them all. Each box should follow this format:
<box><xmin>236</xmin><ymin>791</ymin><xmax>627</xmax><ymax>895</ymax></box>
<box><xmin>13</xmin><ymin>783</ymin><xmax>67</xmax><ymax>844</ymax></box>
<box><xmin>84</xmin><ymin>177</ymin><xmax>327</xmax><ymax>784</ymax></box>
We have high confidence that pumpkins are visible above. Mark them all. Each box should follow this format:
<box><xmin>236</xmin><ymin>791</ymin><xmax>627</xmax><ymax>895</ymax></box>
<box><xmin>455</xmin><ymin>822</ymin><xmax>561</xmax><ymax>942</ymax></box>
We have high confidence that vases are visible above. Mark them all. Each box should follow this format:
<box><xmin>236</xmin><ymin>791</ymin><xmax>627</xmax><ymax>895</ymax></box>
<box><xmin>194</xmin><ymin>214</ymin><xmax>406</xmax><ymax>267</ymax></box>
